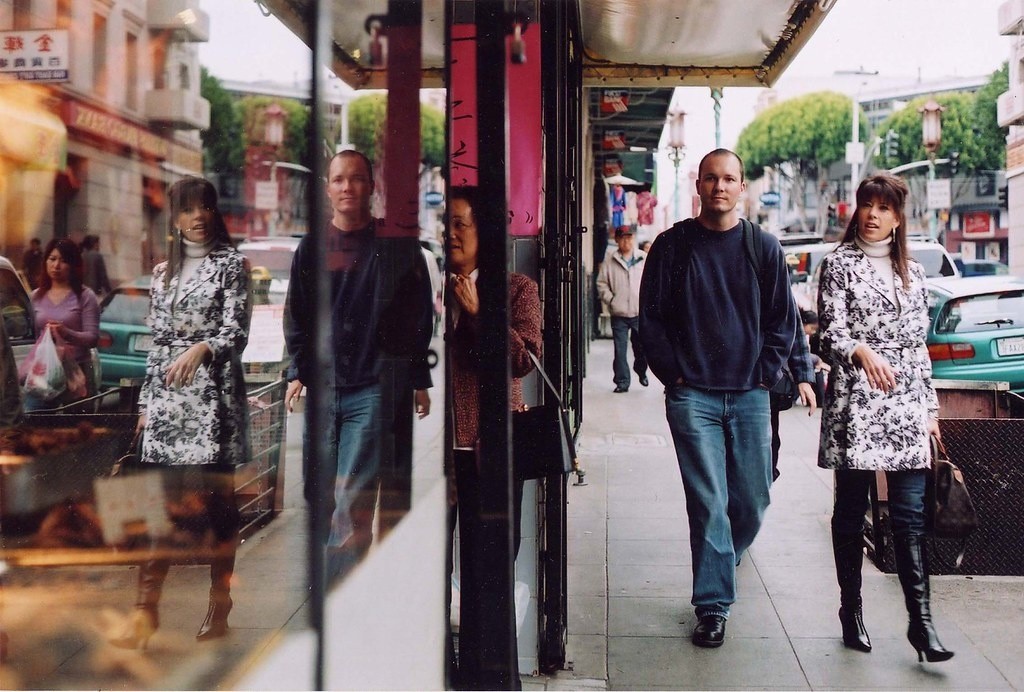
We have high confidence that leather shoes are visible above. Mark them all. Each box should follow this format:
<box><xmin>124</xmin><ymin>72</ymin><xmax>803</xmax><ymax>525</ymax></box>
<box><xmin>692</xmin><ymin>613</ymin><xmax>727</xmax><ymax>648</ymax></box>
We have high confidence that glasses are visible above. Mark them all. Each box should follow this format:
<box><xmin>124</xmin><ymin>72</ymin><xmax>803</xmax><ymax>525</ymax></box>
<box><xmin>448</xmin><ymin>216</ymin><xmax>473</xmax><ymax>233</ymax></box>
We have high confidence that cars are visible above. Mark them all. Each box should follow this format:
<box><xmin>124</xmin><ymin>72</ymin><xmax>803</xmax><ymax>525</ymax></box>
<box><xmin>93</xmin><ymin>273</ymin><xmax>153</xmax><ymax>391</ymax></box>
<box><xmin>925</xmin><ymin>276</ymin><xmax>1024</xmax><ymax>394</ymax></box>
<box><xmin>779</xmin><ymin>230</ymin><xmax>1013</xmax><ymax>312</ymax></box>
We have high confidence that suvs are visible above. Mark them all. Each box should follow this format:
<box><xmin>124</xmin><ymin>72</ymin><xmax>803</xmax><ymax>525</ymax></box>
<box><xmin>0</xmin><ymin>254</ymin><xmax>103</xmax><ymax>414</ymax></box>
<box><xmin>237</xmin><ymin>235</ymin><xmax>306</xmax><ymax>385</ymax></box>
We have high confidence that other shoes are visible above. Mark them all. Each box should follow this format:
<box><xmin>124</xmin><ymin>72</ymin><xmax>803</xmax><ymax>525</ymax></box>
<box><xmin>639</xmin><ymin>376</ymin><xmax>649</xmax><ymax>386</ymax></box>
<box><xmin>612</xmin><ymin>387</ymin><xmax>628</xmax><ymax>393</ymax></box>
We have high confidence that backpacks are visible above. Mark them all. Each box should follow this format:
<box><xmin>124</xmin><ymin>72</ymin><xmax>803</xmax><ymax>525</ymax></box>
<box><xmin>777</xmin><ymin>368</ymin><xmax>792</xmax><ymax>410</ymax></box>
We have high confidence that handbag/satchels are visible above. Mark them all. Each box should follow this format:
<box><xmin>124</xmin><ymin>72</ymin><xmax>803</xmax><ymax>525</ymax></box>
<box><xmin>924</xmin><ymin>434</ymin><xmax>979</xmax><ymax>539</ymax></box>
<box><xmin>509</xmin><ymin>349</ymin><xmax>579</xmax><ymax>483</ymax></box>
<box><xmin>17</xmin><ymin>323</ymin><xmax>87</xmax><ymax>401</ymax></box>
<box><xmin>104</xmin><ymin>429</ymin><xmax>154</xmax><ymax>564</ymax></box>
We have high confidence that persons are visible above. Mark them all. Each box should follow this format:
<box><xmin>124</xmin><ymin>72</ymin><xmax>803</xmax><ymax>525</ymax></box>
<box><xmin>445</xmin><ymin>183</ymin><xmax>542</xmax><ymax>691</ymax></box>
<box><xmin>817</xmin><ymin>176</ymin><xmax>957</xmax><ymax>662</ymax></box>
<box><xmin>283</xmin><ymin>149</ymin><xmax>431</xmax><ymax>594</ymax></box>
<box><xmin>637</xmin><ymin>149</ymin><xmax>816</xmax><ymax>648</ymax></box>
<box><xmin>596</xmin><ymin>225</ymin><xmax>649</xmax><ymax>393</ymax></box>
<box><xmin>134</xmin><ymin>179</ymin><xmax>253</xmax><ymax>639</ymax></box>
<box><xmin>22</xmin><ymin>234</ymin><xmax>115</xmax><ymax>416</ymax></box>
<box><xmin>770</xmin><ymin>308</ymin><xmax>833</xmax><ymax>481</ymax></box>
<box><xmin>640</xmin><ymin>241</ymin><xmax>652</xmax><ymax>255</ymax></box>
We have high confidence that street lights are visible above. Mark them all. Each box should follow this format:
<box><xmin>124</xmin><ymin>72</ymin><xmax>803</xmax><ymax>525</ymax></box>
<box><xmin>917</xmin><ymin>99</ymin><xmax>946</xmax><ymax>241</ymax></box>
<box><xmin>667</xmin><ymin>99</ymin><xmax>688</xmax><ymax>222</ymax></box>
<box><xmin>260</xmin><ymin>97</ymin><xmax>289</xmax><ymax>238</ymax></box>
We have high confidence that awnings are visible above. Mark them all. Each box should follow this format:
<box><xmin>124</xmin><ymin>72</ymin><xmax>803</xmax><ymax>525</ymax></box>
<box><xmin>575</xmin><ymin>0</ymin><xmax>841</xmax><ymax>88</ymax></box>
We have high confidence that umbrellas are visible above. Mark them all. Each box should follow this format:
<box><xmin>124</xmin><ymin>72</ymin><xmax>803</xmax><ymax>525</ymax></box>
<box><xmin>606</xmin><ymin>172</ymin><xmax>644</xmax><ymax>186</ymax></box>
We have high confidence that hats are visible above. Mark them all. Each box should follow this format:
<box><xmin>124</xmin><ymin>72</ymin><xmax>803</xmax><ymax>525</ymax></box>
<box><xmin>614</xmin><ymin>226</ymin><xmax>633</xmax><ymax>238</ymax></box>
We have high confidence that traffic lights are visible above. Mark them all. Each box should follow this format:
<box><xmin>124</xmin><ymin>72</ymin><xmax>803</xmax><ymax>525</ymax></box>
<box><xmin>884</xmin><ymin>129</ymin><xmax>900</xmax><ymax>159</ymax></box>
<box><xmin>827</xmin><ymin>203</ymin><xmax>837</xmax><ymax>228</ymax></box>
<box><xmin>837</xmin><ymin>202</ymin><xmax>846</xmax><ymax>227</ymax></box>
<box><xmin>948</xmin><ymin>149</ymin><xmax>960</xmax><ymax>175</ymax></box>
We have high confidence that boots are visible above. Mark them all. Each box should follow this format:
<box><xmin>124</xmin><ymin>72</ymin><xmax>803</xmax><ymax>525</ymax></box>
<box><xmin>195</xmin><ymin>512</ymin><xmax>241</xmax><ymax>640</ymax></box>
<box><xmin>831</xmin><ymin>506</ymin><xmax>872</xmax><ymax>652</ymax></box>
<box><xmin>892</xmin><ymin>534</ymin><xmax>955</xmax><ymax>662</ymax></box>
<box><xmin>108</xmin><ymin>564</ymin><xmax>171</xmax><ymax>650</ymax></box>
